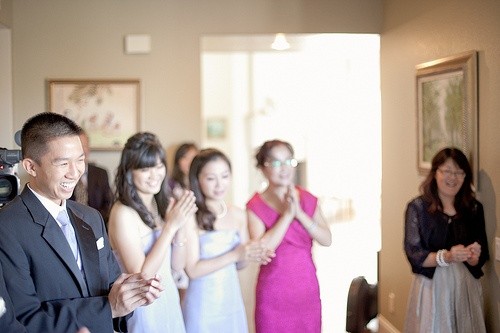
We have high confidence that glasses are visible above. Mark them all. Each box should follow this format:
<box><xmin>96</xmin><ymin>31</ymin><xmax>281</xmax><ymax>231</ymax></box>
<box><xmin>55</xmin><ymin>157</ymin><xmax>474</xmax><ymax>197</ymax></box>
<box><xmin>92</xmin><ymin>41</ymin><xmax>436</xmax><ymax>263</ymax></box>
<box><xmin>271</xmin><ymin>159</ymin><xmax>297</xmax><ymax>168</ymax></box>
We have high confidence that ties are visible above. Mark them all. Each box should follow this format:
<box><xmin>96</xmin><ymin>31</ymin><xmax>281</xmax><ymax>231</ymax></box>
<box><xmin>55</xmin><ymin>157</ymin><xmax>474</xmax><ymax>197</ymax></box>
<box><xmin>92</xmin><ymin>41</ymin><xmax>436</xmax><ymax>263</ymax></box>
<box><xmin>76</xmin><ymin>179</ymin><xmax>88</xmax><ymax>205</ymax></box>
<box><xmin>57</xmin><ymin>210</ymin><xmax>82</xmax><ymax>270</ymax></box>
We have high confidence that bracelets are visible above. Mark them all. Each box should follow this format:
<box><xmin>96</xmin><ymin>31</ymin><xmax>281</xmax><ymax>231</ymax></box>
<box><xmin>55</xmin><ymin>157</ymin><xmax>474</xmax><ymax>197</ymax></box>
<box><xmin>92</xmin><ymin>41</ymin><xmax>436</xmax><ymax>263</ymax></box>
<box><xmin>436</xmin><ymin>249</ymin><xmax>452</xmax><ymax>267</ymax></box>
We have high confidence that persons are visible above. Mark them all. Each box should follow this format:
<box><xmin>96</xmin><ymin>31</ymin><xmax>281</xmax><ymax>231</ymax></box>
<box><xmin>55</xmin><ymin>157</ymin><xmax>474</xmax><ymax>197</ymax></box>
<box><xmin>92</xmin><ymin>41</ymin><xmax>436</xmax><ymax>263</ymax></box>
<box><xmin>180</xmin><ymin>147</ymin><xmax>275</xmax><ymax>333</ymax></box>
<box><xmin>0</xmin><ymin>113</ymin><xmax>165</xmax><ymax>333</ymax></box>
<box><xmin>107</xmin><ymin>132</ymin><xmax>198</xmax><ymax>333</ymax></box>
<box><xmin>246</xmin><ymin>139</ymin><xmax>332</xmax><ymax>333</ymax></box>
<box><xmin>404</xmin><ymin>147</ymin><xmax>491</xmax><ymax>333</ymax></box>
<box><xmin>168</xmin><ymin>142</ymin><xmax>202</xmax><ymax>199</ymax></box>
<box><xmin>66</xmin><ymin>126</ymin><xmax>117</xmax><ymax>236</ymax></box>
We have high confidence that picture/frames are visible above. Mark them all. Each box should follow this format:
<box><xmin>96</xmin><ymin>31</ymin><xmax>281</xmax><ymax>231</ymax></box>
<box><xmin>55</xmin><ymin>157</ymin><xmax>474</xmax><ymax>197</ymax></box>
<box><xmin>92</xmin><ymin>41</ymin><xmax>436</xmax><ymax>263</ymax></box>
<box><xmin>46</xmin><ymin>80</ymin><xmax>142</xmax><ymax>152</ymax></box>
<box><xmin>415</xmin><ymin>51</ymin><xmax>478</xmax><ymax>186</ymax></box>
